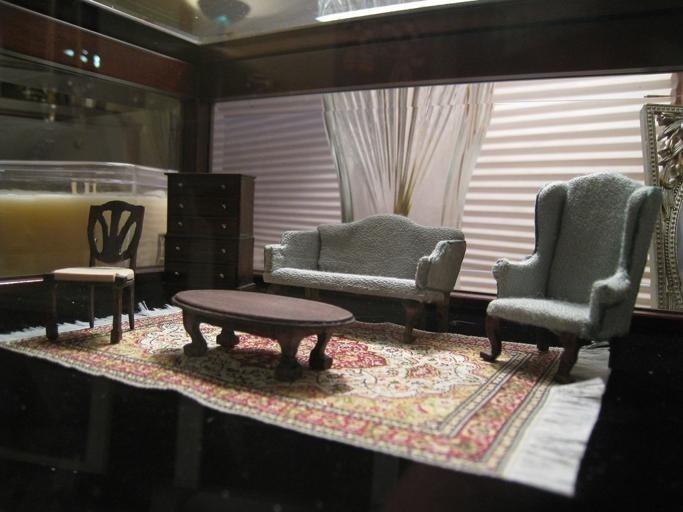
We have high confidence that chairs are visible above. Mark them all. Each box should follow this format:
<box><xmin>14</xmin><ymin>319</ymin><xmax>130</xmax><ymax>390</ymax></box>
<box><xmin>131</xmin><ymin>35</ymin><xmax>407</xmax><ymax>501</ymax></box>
<box><xmin>480</xmin><ymin>171</ymin><xmax>665</xmax><ymax>386</ymax></box>
<box><xmin>40</xmin><ymin>199</ymin><xmax>145</xmax><ymax>345</ymax></box>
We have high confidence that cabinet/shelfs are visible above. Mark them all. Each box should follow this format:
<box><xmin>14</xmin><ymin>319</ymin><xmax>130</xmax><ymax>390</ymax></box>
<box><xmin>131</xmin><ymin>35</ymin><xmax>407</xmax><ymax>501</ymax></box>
<box><xmin>162</xmin><ymin>169</ymin><xmax>259</xmax><ymax>304</ymax></box>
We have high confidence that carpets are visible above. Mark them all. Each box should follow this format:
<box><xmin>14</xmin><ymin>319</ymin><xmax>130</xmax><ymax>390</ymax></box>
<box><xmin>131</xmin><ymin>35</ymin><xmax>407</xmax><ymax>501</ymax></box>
<box><xmin>0</xmin><ymin>302</ymin><xmax>613</xmax><ymax>497</ymax></box>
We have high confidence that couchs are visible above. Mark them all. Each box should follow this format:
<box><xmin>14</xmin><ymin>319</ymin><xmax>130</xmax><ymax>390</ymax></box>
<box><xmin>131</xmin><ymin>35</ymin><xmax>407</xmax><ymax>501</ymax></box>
<box><xmin>261</xmin><ymin>216</ymin><xmax>467</xmax><ymax>346</ymax></box>
